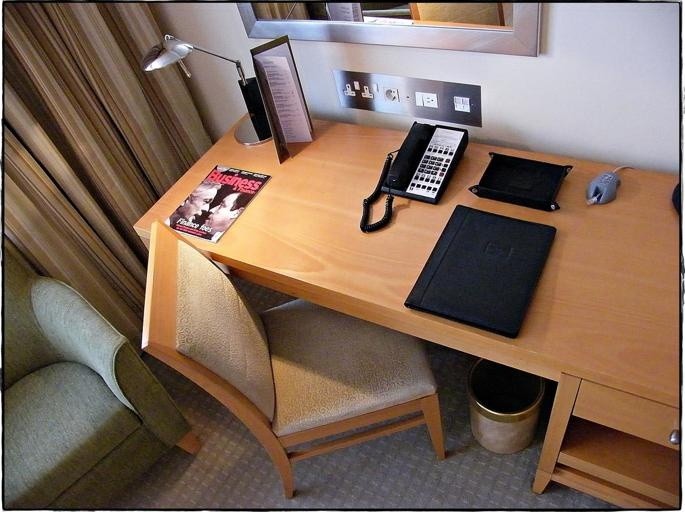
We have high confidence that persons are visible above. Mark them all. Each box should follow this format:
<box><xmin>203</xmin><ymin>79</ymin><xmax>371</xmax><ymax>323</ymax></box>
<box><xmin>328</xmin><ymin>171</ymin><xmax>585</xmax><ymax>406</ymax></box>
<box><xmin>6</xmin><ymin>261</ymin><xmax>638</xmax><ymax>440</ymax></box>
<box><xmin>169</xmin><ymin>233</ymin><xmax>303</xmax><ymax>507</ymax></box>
<box><xmin>201</xmin><ymin>192</ymin><xmax>250</xmax><ymax>233</ymax></box>
<box><xmin>174</xmin><ymin>181</ymin><xmax>223</xmax><ymax>221</ymax></box>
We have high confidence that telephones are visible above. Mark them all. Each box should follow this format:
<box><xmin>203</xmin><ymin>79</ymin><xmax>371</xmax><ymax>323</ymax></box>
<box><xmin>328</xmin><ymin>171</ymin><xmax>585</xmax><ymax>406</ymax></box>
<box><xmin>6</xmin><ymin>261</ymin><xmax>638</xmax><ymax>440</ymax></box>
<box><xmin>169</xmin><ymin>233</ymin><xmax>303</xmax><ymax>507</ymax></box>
<box><xmin>381</xmin><ymin>120</ymin><xmax>470</xmax><ymax>206</ymax></box>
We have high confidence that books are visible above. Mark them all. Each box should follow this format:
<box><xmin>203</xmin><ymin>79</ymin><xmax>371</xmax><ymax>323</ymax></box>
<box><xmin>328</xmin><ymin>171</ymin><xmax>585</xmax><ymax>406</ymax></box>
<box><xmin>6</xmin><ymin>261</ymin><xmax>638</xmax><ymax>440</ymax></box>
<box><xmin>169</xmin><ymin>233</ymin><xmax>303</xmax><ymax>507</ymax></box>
<box><xmin>404</xmin><ymin>204</ymin><xmax>559</xmax><ymax>343</ymax></box>
<box><xmin>160</xmin><ymin>162</ymin><xmax>272</xmax><ymax>244</ymax></box>
<box><xmin>250</xmin><ymin>31</ymin><xmax>317</xmax><ymax>168</ymax></box>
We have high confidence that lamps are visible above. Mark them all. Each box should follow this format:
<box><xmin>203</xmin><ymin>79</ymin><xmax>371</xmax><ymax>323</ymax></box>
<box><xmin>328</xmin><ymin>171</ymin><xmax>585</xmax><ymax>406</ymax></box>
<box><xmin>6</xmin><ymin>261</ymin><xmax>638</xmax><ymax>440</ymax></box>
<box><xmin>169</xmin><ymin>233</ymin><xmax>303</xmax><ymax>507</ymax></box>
<box><xmin>141</xmin><ymin>33</ymin><xmax>270</xmax><ymax>146</ymax></box>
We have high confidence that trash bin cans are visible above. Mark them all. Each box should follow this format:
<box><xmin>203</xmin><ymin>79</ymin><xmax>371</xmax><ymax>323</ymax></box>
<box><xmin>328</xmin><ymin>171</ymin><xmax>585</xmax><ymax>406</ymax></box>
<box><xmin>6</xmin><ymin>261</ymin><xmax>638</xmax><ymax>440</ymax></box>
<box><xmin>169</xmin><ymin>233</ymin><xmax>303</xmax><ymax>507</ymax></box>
<box><xmin>466</xmin><ymin>358</ymin><xmax>546</xmax><ymax>456</ymax></box>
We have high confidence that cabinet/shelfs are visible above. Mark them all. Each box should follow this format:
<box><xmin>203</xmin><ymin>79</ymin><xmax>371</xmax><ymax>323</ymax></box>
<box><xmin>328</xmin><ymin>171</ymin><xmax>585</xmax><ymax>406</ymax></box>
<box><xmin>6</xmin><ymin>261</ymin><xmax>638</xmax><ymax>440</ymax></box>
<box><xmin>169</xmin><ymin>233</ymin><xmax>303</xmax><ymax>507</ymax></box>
<box><xmin>529</xmin><ymin>374</ymin><xmax>680</xmax><ymax>512</ymax></box>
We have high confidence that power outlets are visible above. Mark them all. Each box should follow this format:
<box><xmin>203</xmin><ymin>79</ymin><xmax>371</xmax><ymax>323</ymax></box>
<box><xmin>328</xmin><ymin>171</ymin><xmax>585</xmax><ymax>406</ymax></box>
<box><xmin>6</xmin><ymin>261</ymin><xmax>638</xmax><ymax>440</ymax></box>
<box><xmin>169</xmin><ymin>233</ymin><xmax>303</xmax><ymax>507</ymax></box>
<box><xmin>331</xmin><ymin>69</ymin><xmax>444</xmax><ymax>122</ymax></box>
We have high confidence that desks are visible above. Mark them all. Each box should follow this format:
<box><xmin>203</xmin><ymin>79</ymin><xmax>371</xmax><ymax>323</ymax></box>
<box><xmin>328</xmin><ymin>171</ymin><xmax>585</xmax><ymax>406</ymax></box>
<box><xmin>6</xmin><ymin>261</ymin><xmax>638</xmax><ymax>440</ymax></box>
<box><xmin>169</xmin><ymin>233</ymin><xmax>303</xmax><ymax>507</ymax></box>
<box><xmin>134</xmin><ymin>110</ymin><xmax>679</xmax><ymax>388</ymax></box>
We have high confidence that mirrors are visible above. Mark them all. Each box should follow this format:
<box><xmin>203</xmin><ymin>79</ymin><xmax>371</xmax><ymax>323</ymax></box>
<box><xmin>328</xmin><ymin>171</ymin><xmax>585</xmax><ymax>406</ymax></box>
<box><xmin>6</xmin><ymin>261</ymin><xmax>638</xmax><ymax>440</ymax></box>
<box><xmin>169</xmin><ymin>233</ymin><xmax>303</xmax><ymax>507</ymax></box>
<box><xmin>233</xmin><ymin>0</ymin><xmax>543</xmax><ymax>60</ymax></box>
